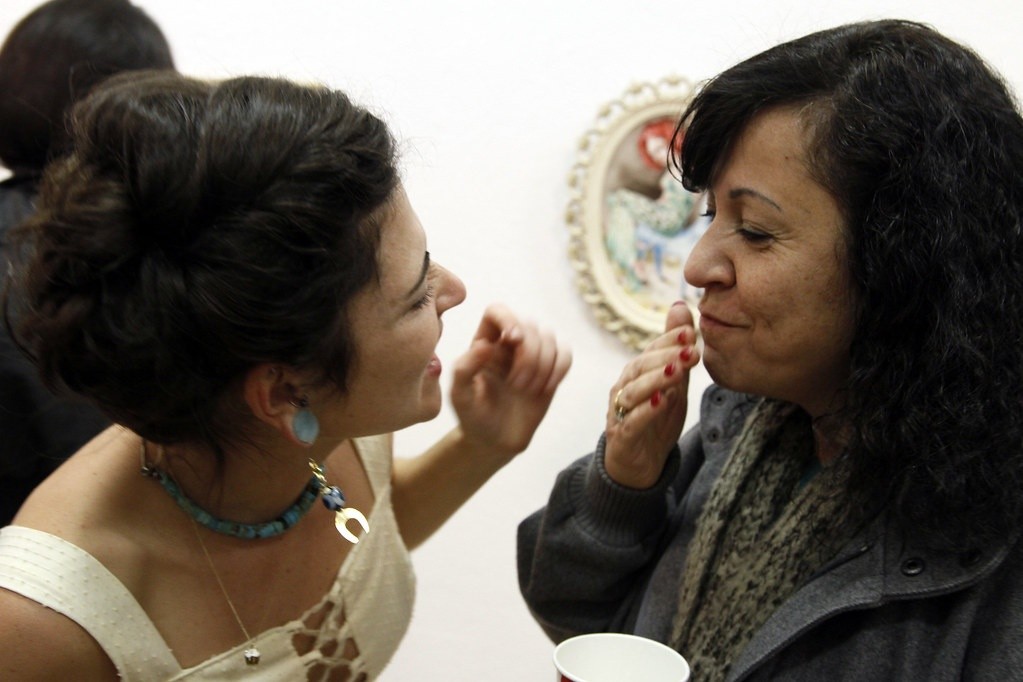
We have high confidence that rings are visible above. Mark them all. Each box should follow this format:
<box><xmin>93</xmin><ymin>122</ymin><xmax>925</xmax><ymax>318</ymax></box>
<box><xmin>614</xmin><ymin>389</ymin><xmax>628</xmax><ymax>423</ymax></box>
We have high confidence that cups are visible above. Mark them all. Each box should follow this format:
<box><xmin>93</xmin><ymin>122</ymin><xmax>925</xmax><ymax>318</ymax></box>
<box><xmin>551</xmin><ymin>632</ymin><xmax>693</xmax><ymax>682</ymax></box>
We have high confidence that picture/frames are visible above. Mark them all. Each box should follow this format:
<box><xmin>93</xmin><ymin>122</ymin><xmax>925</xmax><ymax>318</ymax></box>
<box><xmin>564</xmin><ymin>74</ymin><xmax>713</xmax><ymax>354</ymax></box>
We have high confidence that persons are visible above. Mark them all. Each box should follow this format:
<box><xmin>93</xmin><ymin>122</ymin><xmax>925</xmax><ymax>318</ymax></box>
<box><xmin>0</xmin><ymin>72</ymin><xmax>572</xmax><ymax>682</ymax></box>
<box><xmin>517</xmin><ymin>19</ymin><xmax>1023</xmax><ymax>682</ymax></box>
<box><xmin>0</xmin><ymin>0</ymin><xmax>178</xmax><ymax>529</ymax></box>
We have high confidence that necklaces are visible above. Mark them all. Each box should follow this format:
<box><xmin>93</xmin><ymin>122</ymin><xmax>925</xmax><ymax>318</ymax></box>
<box><xmin>190</xmin><ymin>519</ymin><xmax>306</xmax><ymax>665</ymax></box>
<box><xmin>139</xmin><ymin>438</ymin><xmax>325</xmax><ymax>538</ymax></box>
